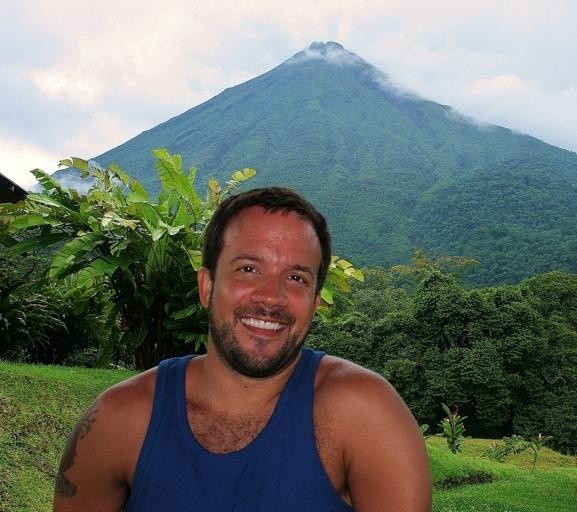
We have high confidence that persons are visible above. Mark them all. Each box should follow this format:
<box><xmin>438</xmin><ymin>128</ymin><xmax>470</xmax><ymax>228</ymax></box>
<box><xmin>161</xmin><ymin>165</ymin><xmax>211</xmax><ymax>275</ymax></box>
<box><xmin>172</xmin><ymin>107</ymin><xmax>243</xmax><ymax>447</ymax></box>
<box><xmin>52</xmin><ymin>185</ymin><xmax>434</xmax><ymax>512</ymax></box>
